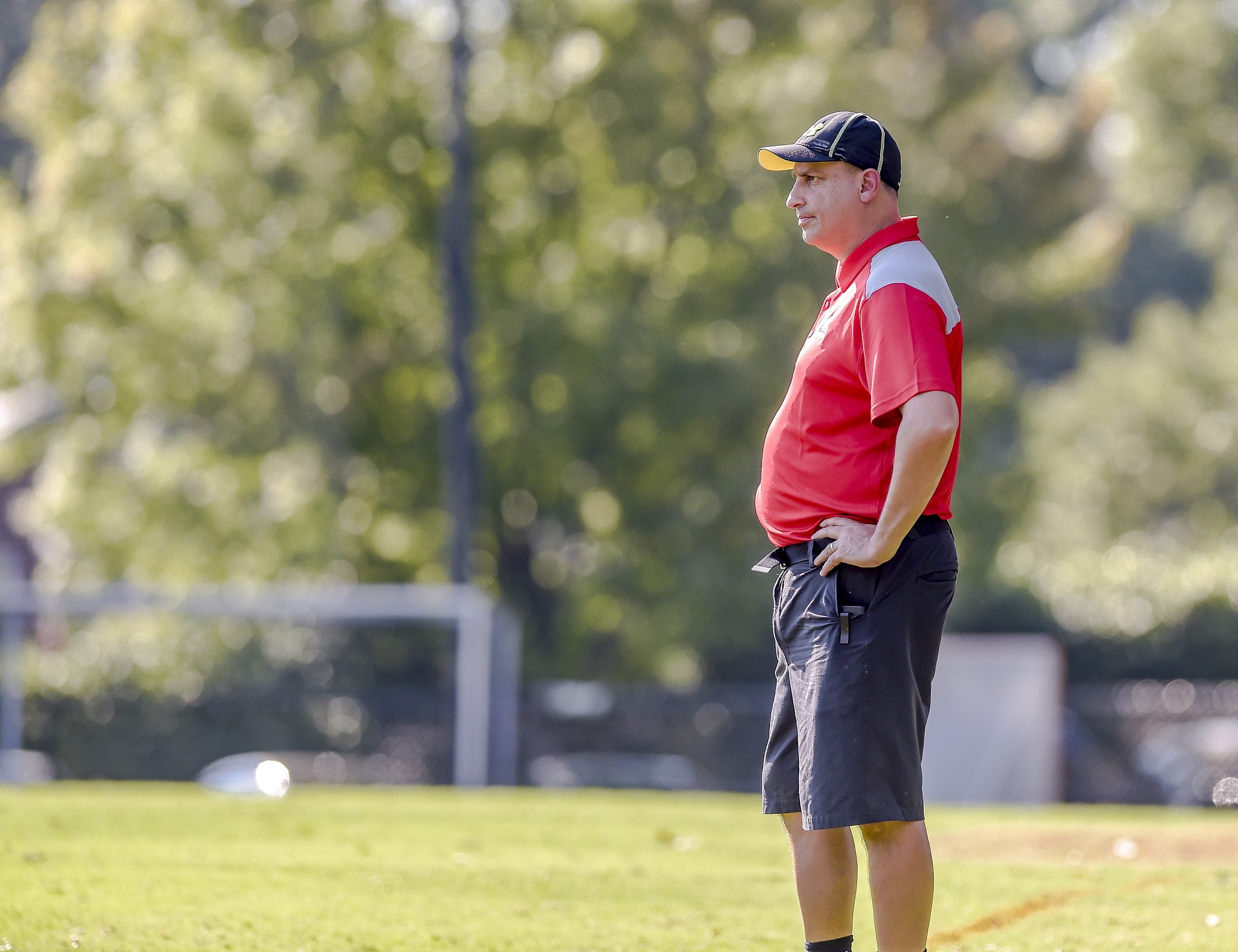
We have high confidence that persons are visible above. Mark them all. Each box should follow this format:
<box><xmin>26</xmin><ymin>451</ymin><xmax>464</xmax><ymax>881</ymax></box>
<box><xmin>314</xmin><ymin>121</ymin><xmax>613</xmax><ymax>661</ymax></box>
<box><xmin>752</xmin><ymin>109</ymin><xmax>964</xmax><ymax>952</ymax></box>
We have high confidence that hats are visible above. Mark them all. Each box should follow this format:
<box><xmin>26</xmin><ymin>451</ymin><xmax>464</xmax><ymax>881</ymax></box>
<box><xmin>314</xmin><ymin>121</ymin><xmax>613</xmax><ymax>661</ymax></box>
<box><xmin>757</xmin><ymin>111</ymin><xmax>901</xmax><ymax>193</ymax></box>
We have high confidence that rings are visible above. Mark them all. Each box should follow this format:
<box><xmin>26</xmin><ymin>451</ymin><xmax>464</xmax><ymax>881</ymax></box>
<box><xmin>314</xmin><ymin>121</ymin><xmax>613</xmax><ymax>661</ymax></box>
<box><xmin>830</xmin><ymin>542</ymin><xmax>837</xmax><ymax>553</ymax></box>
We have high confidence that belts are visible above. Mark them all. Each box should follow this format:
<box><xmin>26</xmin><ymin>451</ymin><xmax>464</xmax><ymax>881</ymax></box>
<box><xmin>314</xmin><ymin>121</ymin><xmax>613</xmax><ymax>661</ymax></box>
<box><xmin>752</xmin><ymin>513</ymin><xmax>950</xmax><ymax>573</ymax></box>
